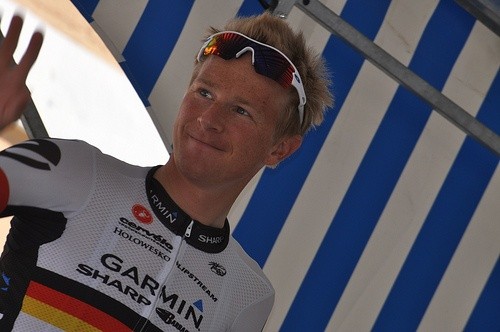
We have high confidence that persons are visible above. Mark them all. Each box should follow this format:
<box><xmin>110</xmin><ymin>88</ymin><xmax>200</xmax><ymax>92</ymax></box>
<box><xmin>0</xmin><ymin>7</ymin><xmax>337</xmax><ymax>331</ymax></box>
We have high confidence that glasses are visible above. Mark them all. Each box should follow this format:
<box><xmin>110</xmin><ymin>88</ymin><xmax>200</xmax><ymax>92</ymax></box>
<box><xmin>194</xmin><ymin>31</ymin><xmax>306</xmax><ymax>124</ymax></box>
